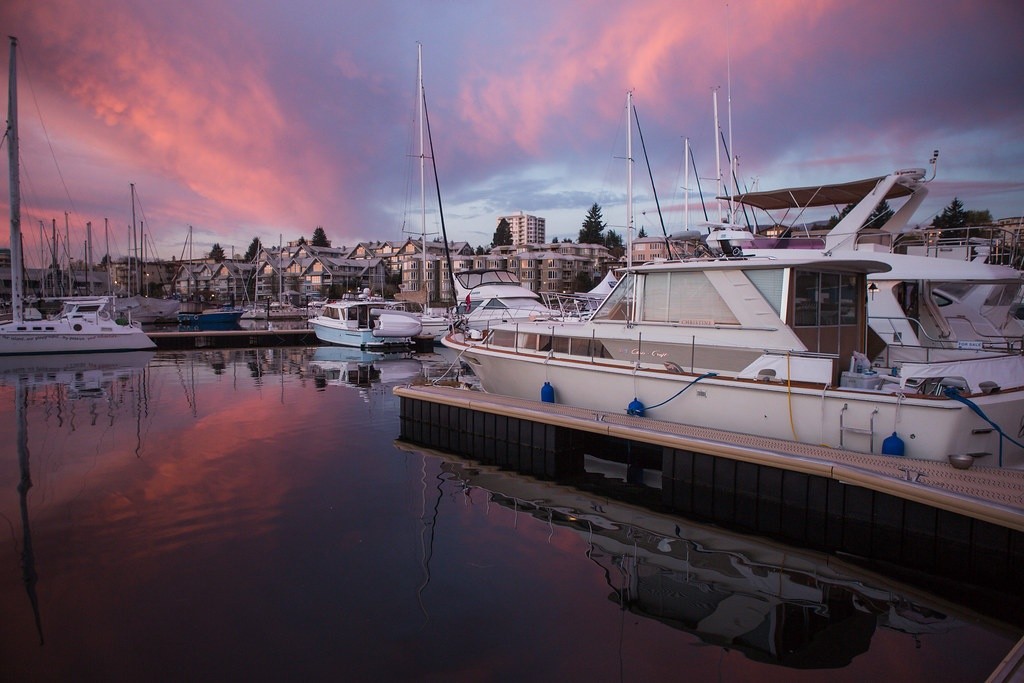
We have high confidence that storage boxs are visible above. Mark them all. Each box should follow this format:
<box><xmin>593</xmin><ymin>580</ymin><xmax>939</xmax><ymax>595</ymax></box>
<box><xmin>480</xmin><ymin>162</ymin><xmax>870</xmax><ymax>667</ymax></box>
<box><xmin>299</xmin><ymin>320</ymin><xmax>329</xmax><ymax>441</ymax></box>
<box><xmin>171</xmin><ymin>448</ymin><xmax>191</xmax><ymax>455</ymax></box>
<box><xmin>838</xmin><ymin>372</ymin><xmax>882</xmax><ymax>389</ymax></box>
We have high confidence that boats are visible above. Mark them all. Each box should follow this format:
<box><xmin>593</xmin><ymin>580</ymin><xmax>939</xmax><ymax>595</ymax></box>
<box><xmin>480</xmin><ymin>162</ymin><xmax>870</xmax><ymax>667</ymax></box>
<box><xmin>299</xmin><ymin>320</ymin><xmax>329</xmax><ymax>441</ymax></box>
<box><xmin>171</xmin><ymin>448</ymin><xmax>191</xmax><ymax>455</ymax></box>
<box><xmin>392</xmin><ymin>83</ymin><xmax>1024</xmax><ymax>568</ymax></box>
<box><xmin>447</xmin><ymin>269</ymin><xmax>563</xmax><ymax>327</ymax></box>
<box><xmin>308</xmin><ymin>299</ymin><xmax>423</xmax><ymax>353</ymax></box>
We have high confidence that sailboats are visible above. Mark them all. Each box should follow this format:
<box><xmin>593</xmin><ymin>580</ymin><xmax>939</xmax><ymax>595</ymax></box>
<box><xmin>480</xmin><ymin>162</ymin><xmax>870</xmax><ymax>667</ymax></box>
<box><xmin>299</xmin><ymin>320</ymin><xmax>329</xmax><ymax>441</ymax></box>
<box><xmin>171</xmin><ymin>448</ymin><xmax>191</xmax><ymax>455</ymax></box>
<box><xmin>1</xmin><ymin>35</ymin><xmax>757</xmax><ymax>356</ymax></box>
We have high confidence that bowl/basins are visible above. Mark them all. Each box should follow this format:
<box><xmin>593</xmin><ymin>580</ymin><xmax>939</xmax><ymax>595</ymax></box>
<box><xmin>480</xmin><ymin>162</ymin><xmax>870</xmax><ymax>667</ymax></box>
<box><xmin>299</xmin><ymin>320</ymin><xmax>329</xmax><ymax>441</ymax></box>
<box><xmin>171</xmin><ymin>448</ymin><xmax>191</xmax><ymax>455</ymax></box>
<box><xmin>948</xmin><ymin>455</ymin><xmax>974</xmax><ymax>469</ymax></box>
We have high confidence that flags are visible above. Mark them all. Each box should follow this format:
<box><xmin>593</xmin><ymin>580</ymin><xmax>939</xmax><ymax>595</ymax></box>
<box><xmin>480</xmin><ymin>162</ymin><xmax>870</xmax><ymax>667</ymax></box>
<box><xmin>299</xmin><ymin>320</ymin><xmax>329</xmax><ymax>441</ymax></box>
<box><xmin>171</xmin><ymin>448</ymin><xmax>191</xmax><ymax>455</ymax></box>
<box><xmin>466</xmin><ymin>295</ymin><xmax>472</xmax><ymax>312</ymax></box>
